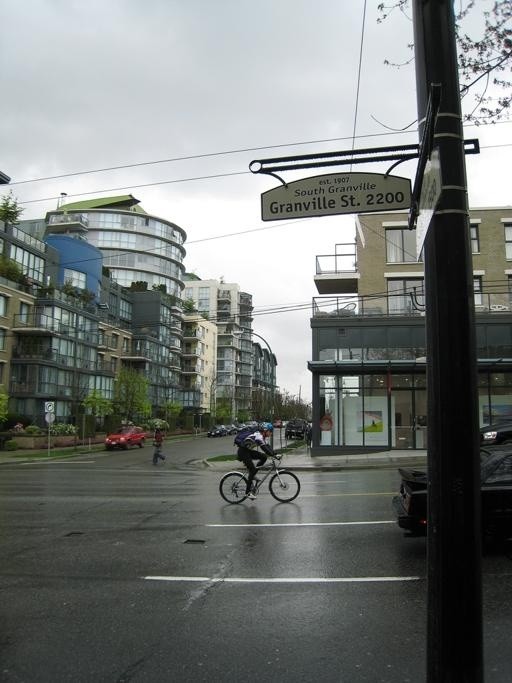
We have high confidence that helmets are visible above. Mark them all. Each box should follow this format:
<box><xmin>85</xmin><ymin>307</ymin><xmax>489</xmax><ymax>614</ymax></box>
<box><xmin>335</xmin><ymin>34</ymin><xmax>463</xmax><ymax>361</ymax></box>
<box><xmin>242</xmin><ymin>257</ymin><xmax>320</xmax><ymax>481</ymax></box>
<box><xmin>261</xmin><ymin>422</ymin><xmax>274</xmax><ymax>432</ymax></box>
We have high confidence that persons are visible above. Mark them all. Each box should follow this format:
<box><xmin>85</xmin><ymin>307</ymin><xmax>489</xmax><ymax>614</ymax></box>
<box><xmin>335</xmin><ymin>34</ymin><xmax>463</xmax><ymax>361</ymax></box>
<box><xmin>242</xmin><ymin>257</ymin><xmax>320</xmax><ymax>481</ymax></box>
<box><xmin>239</xmin><ymin>421</ymin><xmax>283</xmax><ymax>501</ymax></box>
<box><xmin>150</xmin><ymin>428</ymin><xmax>166</xmax><ymax>469</ymax></box>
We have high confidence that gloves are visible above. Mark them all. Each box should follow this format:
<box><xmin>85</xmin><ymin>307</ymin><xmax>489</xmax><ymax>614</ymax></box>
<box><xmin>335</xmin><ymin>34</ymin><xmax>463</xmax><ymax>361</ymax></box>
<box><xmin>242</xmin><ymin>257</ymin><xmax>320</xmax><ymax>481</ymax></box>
<box><xmin>274</xmin><ymin>454</ymin><xmax>282</xmax><ymax>460</ymax></box>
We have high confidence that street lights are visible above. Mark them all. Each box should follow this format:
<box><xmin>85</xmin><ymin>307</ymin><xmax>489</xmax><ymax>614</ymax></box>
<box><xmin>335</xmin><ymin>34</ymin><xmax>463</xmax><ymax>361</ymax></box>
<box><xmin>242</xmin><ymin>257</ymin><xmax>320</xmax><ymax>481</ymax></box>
<box><xmin>209</xmin><ymin>373</ymin><xmax>232</xmax><ymax>431</ymax></box>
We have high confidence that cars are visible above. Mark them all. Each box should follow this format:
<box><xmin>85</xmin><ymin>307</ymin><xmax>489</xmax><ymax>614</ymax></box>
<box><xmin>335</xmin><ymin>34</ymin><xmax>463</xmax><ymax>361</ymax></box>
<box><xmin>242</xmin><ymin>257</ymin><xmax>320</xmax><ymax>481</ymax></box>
<box><xmin>390</xmin><ymin>421</ymin><xmax>512</xmax><ymax>548</ymax></box>
<box><xmin>207</xmin><ymin>420</ymin><xmax>257</xmax><ymax>438</ymax></box>
<box><xmin>273</xmin><ymin>419</ymin><xmax>286</xmax><ymax>428</ymax></box>
<box><xmin>105</xmin><ymin>425</ymin><xmax>149</xmax><ymax>451</ymax></box>
<box><xmin>284</xmin><ymin>419</ymin><xmax>311</xmax><ymax>440</ymax></box>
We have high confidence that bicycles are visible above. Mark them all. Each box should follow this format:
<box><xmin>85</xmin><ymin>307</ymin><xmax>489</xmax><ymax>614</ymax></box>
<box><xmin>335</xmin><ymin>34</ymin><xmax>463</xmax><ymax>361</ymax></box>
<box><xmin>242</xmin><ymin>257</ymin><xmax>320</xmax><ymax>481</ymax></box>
<box><xmin>219</xmin><ymin>454</ymin><xmax>301</xmax><ymax>504</ymax></box>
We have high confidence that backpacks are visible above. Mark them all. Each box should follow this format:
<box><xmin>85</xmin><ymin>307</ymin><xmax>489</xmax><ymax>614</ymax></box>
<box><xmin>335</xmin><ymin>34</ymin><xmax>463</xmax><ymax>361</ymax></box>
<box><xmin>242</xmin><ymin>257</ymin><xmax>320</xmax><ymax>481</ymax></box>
<box><xmin>234</xmin><ymin>429</ymin><xmax>258</xmax><ymax>445</ymax></box>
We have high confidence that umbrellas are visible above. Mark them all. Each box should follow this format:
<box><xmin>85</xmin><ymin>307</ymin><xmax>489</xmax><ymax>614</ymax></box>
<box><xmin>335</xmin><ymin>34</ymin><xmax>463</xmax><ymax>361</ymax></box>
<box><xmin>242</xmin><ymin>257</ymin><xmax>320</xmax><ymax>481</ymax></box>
<box><xmin>146</xmin><ymin>417</ymin><xmax>170</xmax><ymax>428</ymax></box>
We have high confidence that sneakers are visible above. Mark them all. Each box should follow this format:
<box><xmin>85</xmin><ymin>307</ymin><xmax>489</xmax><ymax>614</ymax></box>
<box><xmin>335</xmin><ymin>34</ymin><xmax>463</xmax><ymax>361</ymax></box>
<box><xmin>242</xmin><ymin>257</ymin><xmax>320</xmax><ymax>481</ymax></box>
<box><xmin>254</xmin><ymin>476</ymin><xmax>261</xmax><ymax>481</ymax></box>
<box><xmin>245</xmin><ymin>492</ymin><xmax>257</xmax><ymax>499</ymax></box>
